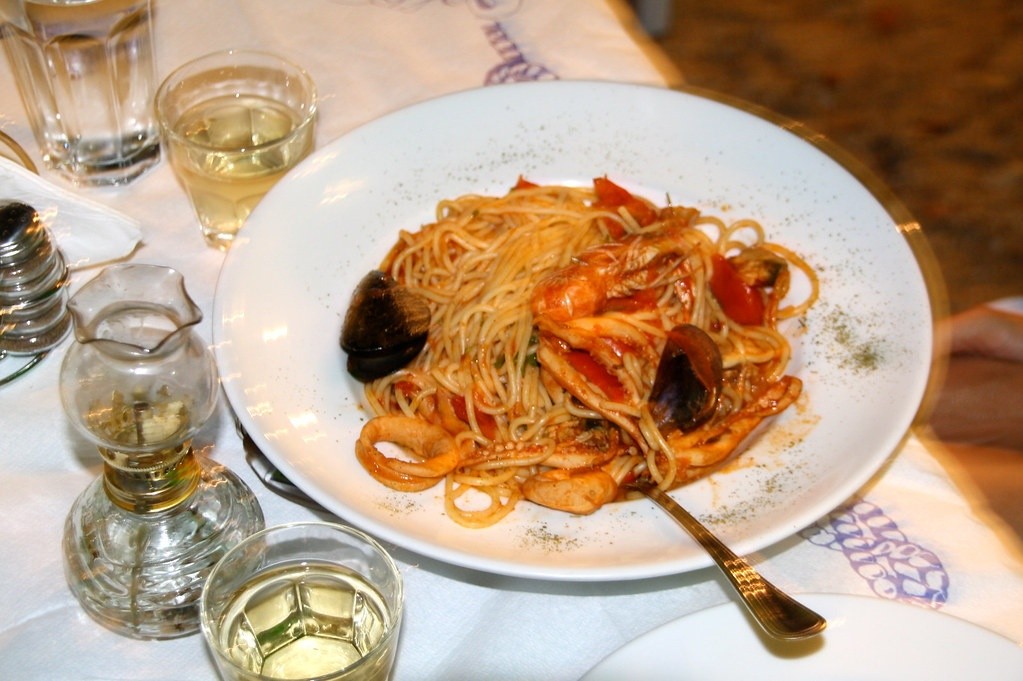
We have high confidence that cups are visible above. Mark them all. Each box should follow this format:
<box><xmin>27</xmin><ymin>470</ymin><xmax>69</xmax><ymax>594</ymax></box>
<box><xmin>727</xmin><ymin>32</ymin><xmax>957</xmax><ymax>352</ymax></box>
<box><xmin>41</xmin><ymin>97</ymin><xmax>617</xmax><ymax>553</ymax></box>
<box><xmin>0</xmin><ymin>0</ymin><xmax>166</xmax><ymax>189</ymax></box>
<box><xmin>198</xmin><ymin>521</ymin><xmax>406</xmax><ymax>681</ymax></box>
<box><xmin>153</xmin><ymin>47</ymin><xmax>319</xmax><ymax>252</ymax></box>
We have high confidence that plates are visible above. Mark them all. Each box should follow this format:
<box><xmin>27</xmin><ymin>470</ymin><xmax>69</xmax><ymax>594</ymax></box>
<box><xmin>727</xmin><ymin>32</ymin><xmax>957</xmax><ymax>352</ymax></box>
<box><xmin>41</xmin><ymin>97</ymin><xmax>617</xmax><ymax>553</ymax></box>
<box><xmin>211</xmin><ymin>79</ymin><xmax>935</xmax><ymax>582</ymax></box>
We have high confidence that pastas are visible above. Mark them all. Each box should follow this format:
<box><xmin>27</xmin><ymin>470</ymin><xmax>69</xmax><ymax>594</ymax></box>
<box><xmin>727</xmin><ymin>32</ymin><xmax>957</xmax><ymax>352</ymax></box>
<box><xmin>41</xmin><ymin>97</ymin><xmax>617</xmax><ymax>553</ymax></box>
<box><xmin>363</xmin><ymin>183</ymin><xmax>818</xmax><ymax>528</ymax></box>
